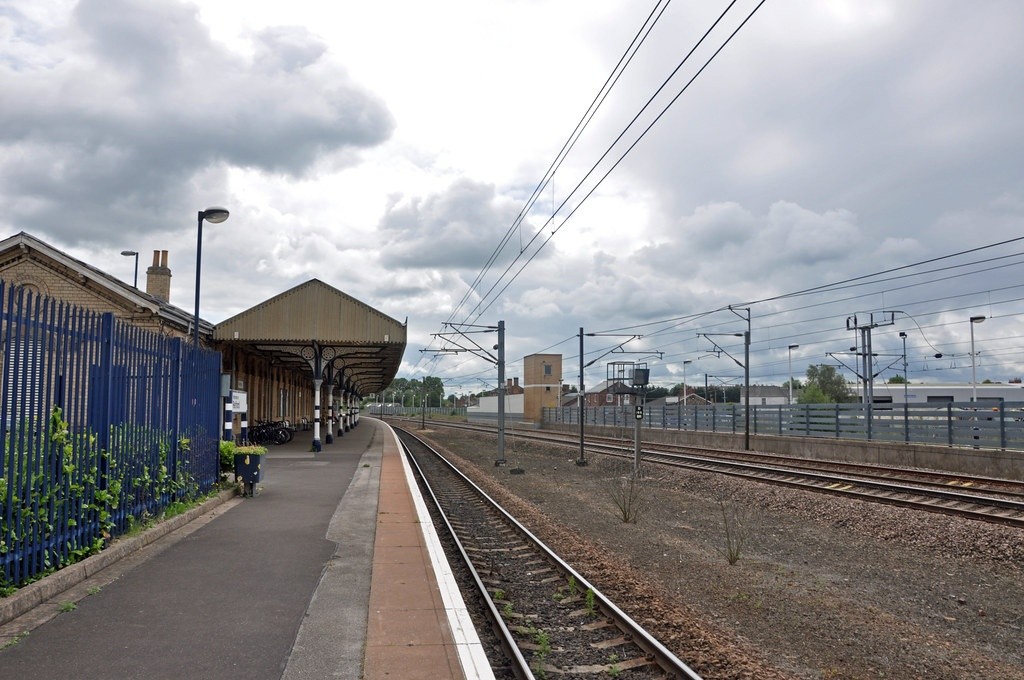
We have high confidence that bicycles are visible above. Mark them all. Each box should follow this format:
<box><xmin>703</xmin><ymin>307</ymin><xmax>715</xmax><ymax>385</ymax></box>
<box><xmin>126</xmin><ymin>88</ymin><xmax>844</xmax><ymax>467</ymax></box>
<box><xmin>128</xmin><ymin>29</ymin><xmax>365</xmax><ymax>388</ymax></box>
<box><xmin>248</xmin><ymin>420</ymin><xmax>295</xmax><ymax>446</ymax></box>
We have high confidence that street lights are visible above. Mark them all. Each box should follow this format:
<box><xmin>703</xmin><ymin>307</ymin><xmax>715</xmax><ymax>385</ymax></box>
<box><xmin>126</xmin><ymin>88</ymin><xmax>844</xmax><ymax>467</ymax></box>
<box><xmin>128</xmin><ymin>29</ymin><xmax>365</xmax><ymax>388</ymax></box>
<box><xmin>899</xmin><ymin>332</ymin><xmax>909</xmax><ymax>444</ymax></box>
<box><xmin>195</xmin><ymin>207</ymin><xmax>229</xmax><ymax>355</ymax></box>
<box><xmin>454</xmin><ymin>393</ymin><xmax>458</xmax><ymax>408</ymax></box>
<box><xmin>402</xmin><ymin>395</ymin><xmax>405</xmax><ymax>412</ymax></box>
<box><xmin>482</xmin><ymin>390</ymin><xmax>485</xmax><ymax>396</ymax></box>
<box><xmin>122</xmin><ymin>249</ymin><xmax>138</xmax><ymax>291</ymax></box>
<box><xmin>684</xmin><ymin>358</ymin><xmax>692</xmax><ymax>430</ymax></box>
<box><xmin>425</xmin><ymin>394</ymin><xmax>428</xmax><ymax>414</ymax></box>
<box><xmin>376</xmin><ymin>394</ymin><xmax>379</xmax><ymax>411</ymax></box>
<box><xmin>788</xmin><ymin>343</ymin><xmax>799</xmax><ymax>434</ymax></box>
<box><xmin>393</xmin><ymin>395</ymin><xmax>396</xmax><ymax>413</ymax></box>
<box><xmin>439</xmin><ymin>393</ymin><xmax>443</xmax><ymax>413</ymax></box>
<box><xmin>559</xmin><ymin>378</ymin><xmax>564</xmax><ymax>421</ymax></box>
<box><xmin>971</xmin><ymin>315</ymin><xmax>986</xmax><ymax>449</ymax></box>
<box><xmin>468</xmin><ymin>391</ymin><xmax>472</xmax><ymax>407</ymax></box>
<box><xmin>413</xmin><ymin>395</ymin><xmax>416</xmax><ymax>411</ymax></box>
<box><xmin>577</xmin><ymin>375</ymin><xmax>581</xmax><ymax>424</ymax></box>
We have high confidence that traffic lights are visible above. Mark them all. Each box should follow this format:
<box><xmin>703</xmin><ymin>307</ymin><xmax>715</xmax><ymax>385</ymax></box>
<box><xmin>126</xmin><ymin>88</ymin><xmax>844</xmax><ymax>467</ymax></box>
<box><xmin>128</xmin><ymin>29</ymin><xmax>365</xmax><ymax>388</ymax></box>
<box><xmin>629</xmin><ymin>370</ymin><xmax>651</xmax><ymax>386</ymax></box>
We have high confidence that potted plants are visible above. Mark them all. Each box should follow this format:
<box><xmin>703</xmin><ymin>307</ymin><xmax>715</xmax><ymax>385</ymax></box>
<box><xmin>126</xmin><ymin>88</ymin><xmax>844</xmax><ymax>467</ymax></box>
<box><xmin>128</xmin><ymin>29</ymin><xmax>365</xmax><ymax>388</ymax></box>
<box><xmin>232</xmin><ymin>445</ymin><xmax>268</xmax><ymax>483</ymax></box>
